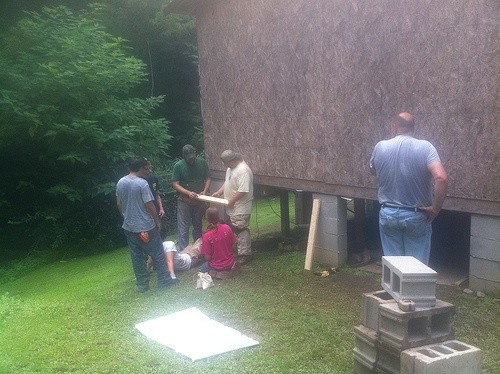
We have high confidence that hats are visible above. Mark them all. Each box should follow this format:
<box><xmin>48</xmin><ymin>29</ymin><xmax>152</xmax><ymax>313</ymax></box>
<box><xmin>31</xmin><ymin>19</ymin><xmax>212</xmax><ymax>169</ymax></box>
<box><xmin>221</xmin><ymin>150</ymin><xmax>239</xmax><ymax>162</ymax></box>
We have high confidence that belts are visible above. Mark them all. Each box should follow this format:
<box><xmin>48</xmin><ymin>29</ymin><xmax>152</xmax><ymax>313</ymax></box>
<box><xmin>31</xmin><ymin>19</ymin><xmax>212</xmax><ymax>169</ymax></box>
<box><xmin>384</xmin><ymin>205</ymin><xmax>425</xmax><ymax>212</ymax></box>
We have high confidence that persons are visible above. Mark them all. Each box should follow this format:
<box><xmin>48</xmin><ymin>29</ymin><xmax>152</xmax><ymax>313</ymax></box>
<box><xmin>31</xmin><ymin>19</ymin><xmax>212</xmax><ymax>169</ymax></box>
<box><xmin>172</xmin><ymin>144</ymin><xmax>211</xmax><ymax>252</ymax></box>
<box><xmin>200</xmin><ymin>208</ymin><xmax>238</xmax><ymax>279</ymax></box>
<box><xmin>116</xmin><ymin>158</ymin><xmax>180</xmax><ymax>293</ymax></box>
<box><xmin>368</xmin><ymin>112</ymin><xmax>448</xmax><ymax>268</ymax></box>
<box><xmin>140</xmin><ymin>161</ymin><xmax>165</xmax><ymax>273</ymax></box>
<box><xmin>160</xmin><ymin>227</ymin><xmax>207</xmax><ymax>280</ymax></box>
<box><xmin>212</xmin><ymin>150</ymin><xmax>253</xmax><ymax>265</ymax></box>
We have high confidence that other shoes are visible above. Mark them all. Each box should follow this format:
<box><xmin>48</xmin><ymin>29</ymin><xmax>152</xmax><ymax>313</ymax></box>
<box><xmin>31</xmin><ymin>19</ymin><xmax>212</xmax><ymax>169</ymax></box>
<box><xmin>238</xmin><ymin>254</ymin><xmax>250</xmax><ymax>265</ymax></box>
<box><xmin>209</xmin><ymin>269</ymin><xmax>231</xmax><ymax>279</ymax></box>
<box><xmin>171</xmin><ymin>278</ymin><xmax>179</xmax><ymax>284</ymax></box>
